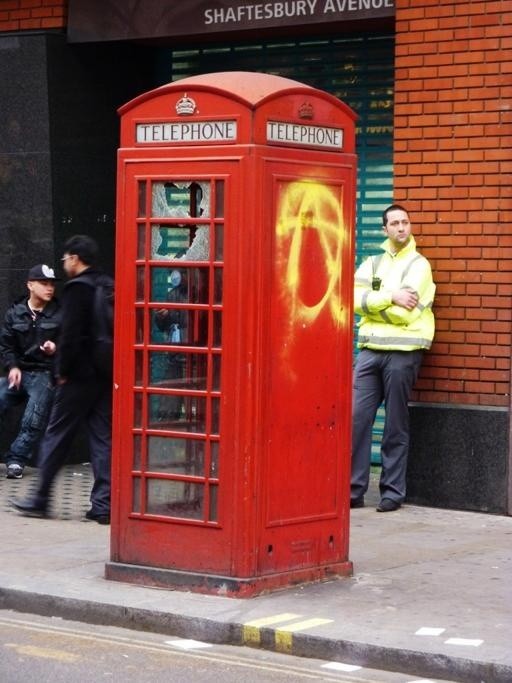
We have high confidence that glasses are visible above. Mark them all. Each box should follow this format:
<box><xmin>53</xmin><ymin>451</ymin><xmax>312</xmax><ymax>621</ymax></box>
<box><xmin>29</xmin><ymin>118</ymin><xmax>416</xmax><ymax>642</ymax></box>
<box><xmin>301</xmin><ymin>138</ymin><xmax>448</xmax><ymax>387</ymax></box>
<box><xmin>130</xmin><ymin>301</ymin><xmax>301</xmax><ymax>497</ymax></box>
<box><xmin>60</xmin><ymin>255</ymin><xmax>79</xmax><ymax>261</ymax></box>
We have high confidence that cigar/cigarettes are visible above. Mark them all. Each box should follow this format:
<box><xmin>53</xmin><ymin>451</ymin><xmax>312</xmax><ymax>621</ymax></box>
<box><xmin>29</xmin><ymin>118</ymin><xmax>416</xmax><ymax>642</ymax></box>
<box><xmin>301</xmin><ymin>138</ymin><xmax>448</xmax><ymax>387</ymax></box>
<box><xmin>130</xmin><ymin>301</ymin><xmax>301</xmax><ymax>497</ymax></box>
<box><xmin>8</xmin><ymin>381</ymin><xmax>16</xmax><ymax>387</ymax></box>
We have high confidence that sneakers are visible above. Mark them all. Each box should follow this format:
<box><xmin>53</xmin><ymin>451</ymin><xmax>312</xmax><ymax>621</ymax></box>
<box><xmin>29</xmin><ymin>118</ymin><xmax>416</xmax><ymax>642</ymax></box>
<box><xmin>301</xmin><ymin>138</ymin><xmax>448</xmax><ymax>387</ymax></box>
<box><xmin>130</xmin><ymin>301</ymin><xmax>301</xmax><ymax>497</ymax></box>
<box><xmin>86</xmin><ymin>510</ymin><xmax>111</xmax><ymax>524</ymax></box>
<box><xmin>11</xmin><ymin>500</ymin><xmax>44</xmax><ymax>518</ymax></box>
<box><xmin>5</xmin><ymin>462</ymin><xmax>24</xmax><ymax>479</ymax></box>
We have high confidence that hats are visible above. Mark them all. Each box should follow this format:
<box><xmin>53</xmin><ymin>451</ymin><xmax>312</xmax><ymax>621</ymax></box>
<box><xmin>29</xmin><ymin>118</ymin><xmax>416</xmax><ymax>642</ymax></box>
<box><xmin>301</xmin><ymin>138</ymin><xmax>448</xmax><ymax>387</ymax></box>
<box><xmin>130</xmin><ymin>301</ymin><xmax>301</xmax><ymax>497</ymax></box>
<box><xmin>28</xmin><ymin>264</ymin><xmax>62</xmax><ymax>280</ymax></box>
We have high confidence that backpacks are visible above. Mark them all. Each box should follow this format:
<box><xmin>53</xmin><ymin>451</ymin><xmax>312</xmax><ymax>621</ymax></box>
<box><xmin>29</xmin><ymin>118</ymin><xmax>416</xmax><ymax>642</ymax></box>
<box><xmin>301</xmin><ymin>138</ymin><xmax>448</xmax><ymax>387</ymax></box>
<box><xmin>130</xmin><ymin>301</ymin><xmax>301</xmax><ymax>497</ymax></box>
<box><xmin>63</xmin><ymin>275</ymin><xmax>114</xmax><ymax>374</ymax></box>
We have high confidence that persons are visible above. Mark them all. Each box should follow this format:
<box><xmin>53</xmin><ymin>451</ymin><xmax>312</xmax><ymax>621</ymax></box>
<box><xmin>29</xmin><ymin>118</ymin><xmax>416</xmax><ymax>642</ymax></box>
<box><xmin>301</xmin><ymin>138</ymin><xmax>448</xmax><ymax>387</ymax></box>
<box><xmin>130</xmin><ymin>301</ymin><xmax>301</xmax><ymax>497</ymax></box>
<box><xmin>154</xmin><ymin>266</ymin><xmax>189</xmax><ymax>424</ymax></box>
<box><xmin>8</xmin><ymin>233</ymin><xmax>115</xmax><ymax>523</ymax></box>
<box><xmin>349</xmin><ymin>204</ymin><xmax>435</xmax><ymax>512</ymax></box>
<box><xmin>0</xmin><ymin>263</ymin><xmax>62</xmax><ymax>479</ymax></box>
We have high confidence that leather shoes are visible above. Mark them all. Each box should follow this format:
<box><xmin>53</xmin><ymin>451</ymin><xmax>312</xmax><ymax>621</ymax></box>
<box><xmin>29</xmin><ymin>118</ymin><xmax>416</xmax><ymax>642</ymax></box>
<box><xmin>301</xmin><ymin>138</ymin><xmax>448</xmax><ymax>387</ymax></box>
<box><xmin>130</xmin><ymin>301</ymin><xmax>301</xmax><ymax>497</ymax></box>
<box><xmin>376</xmin><ymin>498</ymin><xmax>400</xmax><ymax>511</ymax></box>
<box><xmin>349</xmin><ymin>496</ymin><xmax>364</xmax><ymax>507</ymax></box>
